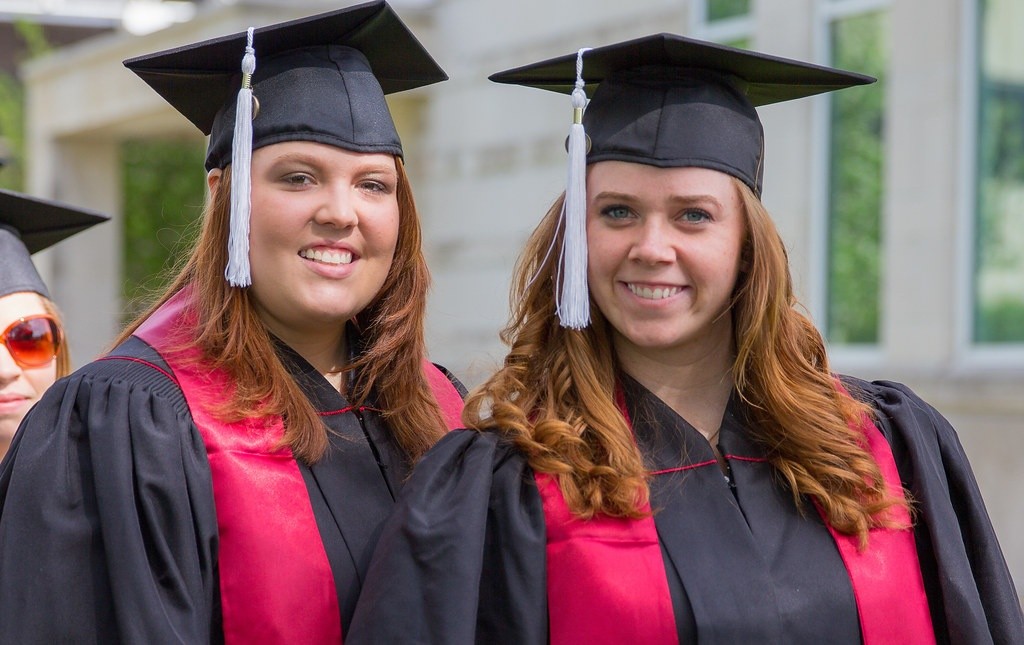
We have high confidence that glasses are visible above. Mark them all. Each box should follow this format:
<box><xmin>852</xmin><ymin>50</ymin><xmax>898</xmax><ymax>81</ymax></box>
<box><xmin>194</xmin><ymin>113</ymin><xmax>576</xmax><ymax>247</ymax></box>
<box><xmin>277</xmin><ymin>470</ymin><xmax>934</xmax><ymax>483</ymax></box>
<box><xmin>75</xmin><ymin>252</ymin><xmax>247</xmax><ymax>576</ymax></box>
<box><xmin>0</xmin><ymin>314</ymin><xmax>61</xmax><ymax>366</ymax></box>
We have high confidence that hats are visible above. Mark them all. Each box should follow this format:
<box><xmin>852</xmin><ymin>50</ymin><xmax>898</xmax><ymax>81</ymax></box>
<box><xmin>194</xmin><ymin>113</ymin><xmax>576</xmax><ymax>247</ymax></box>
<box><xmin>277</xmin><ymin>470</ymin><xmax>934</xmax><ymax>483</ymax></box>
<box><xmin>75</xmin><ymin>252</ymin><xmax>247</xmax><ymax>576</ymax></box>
<box><xmin>123</xmin><ymin>0</ymin><xmax>449</xmax><ymax>285</ymax></box>
<box><xmin>487</xmin><ymin>32</ymin><xmax>876</xmax><ymax>331</ymax></box>
<box><xmin>0</xmin><ymin>188</ymin><xmax>112</xmax><ymax>299</ymax></box>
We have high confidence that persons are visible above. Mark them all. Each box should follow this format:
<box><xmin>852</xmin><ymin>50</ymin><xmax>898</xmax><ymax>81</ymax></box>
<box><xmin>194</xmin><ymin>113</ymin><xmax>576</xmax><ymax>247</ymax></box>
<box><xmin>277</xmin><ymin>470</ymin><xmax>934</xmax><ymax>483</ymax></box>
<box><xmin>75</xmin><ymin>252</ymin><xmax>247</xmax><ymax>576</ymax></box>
<box><xmin>0</xmin><ymin>189</ymin><xmax>108</xmax><ymax>475</ymax></box>
<box><xmin>345</xmin><ymin>31</ymin><xmax>1024</xmax><ymax>644</ymax></box>
<box><xmin>0</xmin><ymin>0</ymin><xmax>481</xmax><ymax>645</ymax></box>
